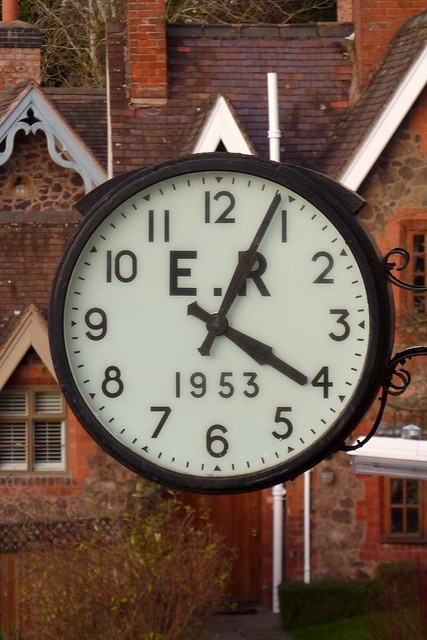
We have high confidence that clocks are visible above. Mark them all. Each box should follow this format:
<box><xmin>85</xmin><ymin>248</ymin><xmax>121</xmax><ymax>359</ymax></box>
<box><xmin>50</xmin><ymin>152</ymin><xmax>397</xmax><ymax>497</ymax></box>
